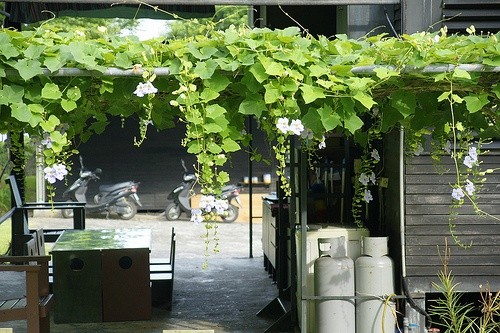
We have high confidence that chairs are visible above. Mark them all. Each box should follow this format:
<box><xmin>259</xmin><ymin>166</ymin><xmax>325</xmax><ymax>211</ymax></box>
<box><xmin>0</xmin><ymin>253</ymin><xmax>52</xmax><ymax>333</ymax></box>
<box><xmin>4</xmin><ymin>174</ymin><xmax>86</xmax><ymax>247</ymax></box>
<box><xmin>150</xmin><ymin>236</ymin><xmax>176</xmax><ymax>313</ymax></box>
<box><xmin>150</xmin><ymin>232</ymin><xmax>176</xmax><ymax>273</ymax></box>
<box><xmin>149</xmin><ymin>226</ymin><xmax>174</xmax><ymax>265</ymax></box>
<box><xmin>36</xmin><ymin>228</ymin><xmax>53</xmax><ymax>267</ymax></box>
<box><xmin>25</xmin><ymin>238</ymin><xmax>54</xmax><ymax>293</ymax></box>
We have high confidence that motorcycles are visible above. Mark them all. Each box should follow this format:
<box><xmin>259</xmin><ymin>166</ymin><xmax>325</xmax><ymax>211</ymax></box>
<box><xmin>164</xmin><ymin>153</ymin><xmax>243</xmax><ymax>224</ymax></box>
<box><xmin>60</xmin><ymin>156</ymin><xmax>142</xmax><ymax>220</ymax></box>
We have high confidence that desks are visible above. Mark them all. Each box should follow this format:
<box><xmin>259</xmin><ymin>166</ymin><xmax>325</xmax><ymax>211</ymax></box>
<box><xmin>48</xmin><ymin>226</ymin><xmax>153</xmax><ymax>325</ymax></box>
